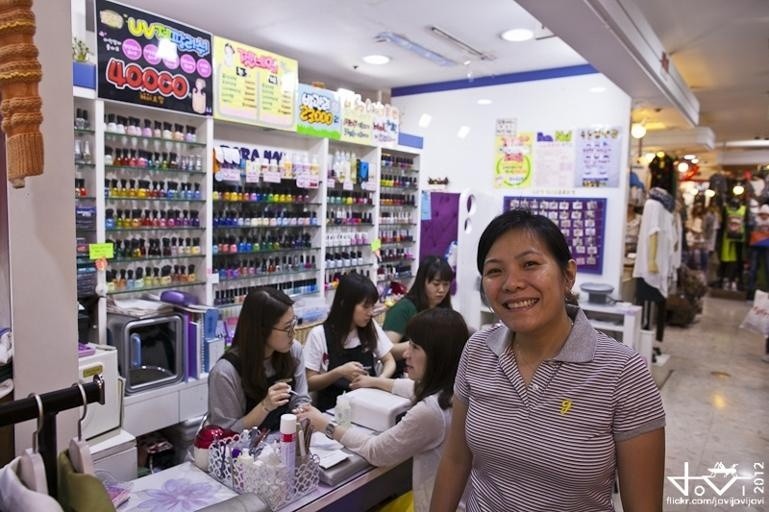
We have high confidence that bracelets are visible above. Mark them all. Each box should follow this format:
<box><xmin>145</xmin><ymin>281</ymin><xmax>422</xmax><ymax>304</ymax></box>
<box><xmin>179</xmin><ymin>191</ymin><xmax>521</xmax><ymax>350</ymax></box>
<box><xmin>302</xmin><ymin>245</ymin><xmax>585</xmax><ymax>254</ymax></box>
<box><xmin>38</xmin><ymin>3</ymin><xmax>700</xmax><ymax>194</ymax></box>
<box><xmin>325</xmin><ymin>418</ymin><xmax>341</xmax><ymax>440</ymax></box>
<box><xmin>261</xmin><ymin>400</ymin><xmax>271</xmax><ymax>413</ymax></box>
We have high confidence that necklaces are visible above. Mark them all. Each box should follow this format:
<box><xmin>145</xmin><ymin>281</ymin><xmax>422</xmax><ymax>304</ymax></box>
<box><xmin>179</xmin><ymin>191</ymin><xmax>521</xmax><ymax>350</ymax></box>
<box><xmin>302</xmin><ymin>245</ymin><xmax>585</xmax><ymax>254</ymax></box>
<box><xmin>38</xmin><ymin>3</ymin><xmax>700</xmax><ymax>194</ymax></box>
<box><xmin>514</xmin><ymin>317</ymin><xmax>574</xmax><ymax>374</ymax></box>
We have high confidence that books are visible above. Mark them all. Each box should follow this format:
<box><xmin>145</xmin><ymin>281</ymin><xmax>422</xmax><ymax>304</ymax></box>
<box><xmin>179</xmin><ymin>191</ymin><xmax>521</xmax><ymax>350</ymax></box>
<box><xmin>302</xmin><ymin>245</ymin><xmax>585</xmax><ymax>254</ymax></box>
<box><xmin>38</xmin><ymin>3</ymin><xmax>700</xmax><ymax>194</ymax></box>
<box><xmin>185</xmin><ymin>292</ymin><xmax>225</xmax><ymax>384</ymax></box>
<box><xmin>105</xmin><ymin>298</ymin><xmax>176</xmax><ymax>317</ymax></box>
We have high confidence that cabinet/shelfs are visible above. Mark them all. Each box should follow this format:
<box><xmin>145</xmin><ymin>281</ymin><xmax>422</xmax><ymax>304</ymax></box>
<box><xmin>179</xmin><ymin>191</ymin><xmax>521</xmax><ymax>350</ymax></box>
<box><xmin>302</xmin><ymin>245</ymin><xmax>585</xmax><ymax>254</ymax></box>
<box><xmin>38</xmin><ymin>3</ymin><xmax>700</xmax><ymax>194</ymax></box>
<box><xmin>479</xmin><ymin>290</ymin><xmax>643</xmax><ymax>352</ymax></box>
<box><xmin>74</xmin><ymin>87</ymin><xmax>424</xmax><ymax>437</ymax></box>
<box><xmin>87</xmin><ymin>381</ymin><xmax>208</xmax><ymax>483</ymax></box>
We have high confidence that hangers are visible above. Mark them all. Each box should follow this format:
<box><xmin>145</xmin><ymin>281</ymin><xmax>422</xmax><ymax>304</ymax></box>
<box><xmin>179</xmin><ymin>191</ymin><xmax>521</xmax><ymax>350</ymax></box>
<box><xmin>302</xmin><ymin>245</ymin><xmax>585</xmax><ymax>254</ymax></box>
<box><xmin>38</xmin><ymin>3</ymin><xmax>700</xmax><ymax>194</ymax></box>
<box><xmin>18</xmin><ymin>393</ymin><xmax>49</xmax><ymax>496</ymax></box>
<box><xmin>68</xmin><ymin>382</ymin><xmax>95</xmax><ymax>476</ymax></box>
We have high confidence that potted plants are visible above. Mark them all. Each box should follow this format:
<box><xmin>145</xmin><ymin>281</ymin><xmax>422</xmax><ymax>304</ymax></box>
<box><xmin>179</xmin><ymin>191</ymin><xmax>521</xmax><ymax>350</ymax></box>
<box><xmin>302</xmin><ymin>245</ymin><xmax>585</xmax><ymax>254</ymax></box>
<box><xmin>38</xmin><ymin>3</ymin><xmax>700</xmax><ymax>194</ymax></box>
<box><xmin>72</xmin><ymin>37</ymin><xmax>96</xmax><ymax>88</ymax></box>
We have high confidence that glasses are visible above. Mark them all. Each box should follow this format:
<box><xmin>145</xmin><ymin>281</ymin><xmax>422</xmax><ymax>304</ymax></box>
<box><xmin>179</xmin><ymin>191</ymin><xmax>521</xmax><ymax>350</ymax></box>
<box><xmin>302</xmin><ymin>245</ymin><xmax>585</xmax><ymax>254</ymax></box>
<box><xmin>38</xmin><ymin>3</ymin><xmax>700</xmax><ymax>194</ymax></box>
<box><xmin>271</xmin><ymin>320</ymin><xmax>297</xmax><ymax>338</ymax></box>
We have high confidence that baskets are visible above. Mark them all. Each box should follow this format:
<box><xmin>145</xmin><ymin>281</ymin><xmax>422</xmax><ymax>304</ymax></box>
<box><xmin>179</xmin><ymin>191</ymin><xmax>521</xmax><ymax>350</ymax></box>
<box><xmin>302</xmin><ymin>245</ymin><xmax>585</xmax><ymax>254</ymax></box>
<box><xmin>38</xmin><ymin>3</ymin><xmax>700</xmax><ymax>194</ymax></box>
<box><xmin>208</xmin><ymin>428</ymin><xmax>322</xmax><ymax>512</ymax></box>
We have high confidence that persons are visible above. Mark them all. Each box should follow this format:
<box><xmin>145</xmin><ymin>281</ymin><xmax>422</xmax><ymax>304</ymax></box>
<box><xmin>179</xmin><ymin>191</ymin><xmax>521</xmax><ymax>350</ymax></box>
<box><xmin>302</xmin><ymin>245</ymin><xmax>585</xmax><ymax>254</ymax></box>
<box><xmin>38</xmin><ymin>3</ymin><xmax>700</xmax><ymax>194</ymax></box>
<box><xmin>382</xmin><ymin>254</ymin><xmax>454</xmax><ymax>380</ymax></box>
<box><xmin>625</xmin><ymin>193</ymin><xmax>641</xmax><ymax>269</ymax></box>
<box><xmin>207</xmin><ymin>286</ymin><xmax>313</xmax><ymax>440</ymax></box>
<box><xmin>634</xmin><ymin>152</ymin><xmax>769</xmax><ymax>364</ymax></box>
<box><xmin>302</xmin><ymin>271</ymin><xmax>397</xmax><ymax>413</ymax></box>
<box><xmin>295</xmin><ymin>307</ymin><xmax>473</xmax><ymax>511</ymax></box>
<box><xmin>429</xmin><ymin>207</ymin><xmax>667</xmax><ymax>512</ymax></box>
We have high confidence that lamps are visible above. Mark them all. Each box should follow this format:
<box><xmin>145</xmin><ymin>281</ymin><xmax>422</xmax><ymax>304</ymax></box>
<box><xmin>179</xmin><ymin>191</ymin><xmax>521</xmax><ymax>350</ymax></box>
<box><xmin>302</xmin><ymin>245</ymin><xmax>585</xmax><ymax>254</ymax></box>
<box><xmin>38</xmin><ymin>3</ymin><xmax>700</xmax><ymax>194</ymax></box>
<box><xmin>431</xmin><ymin>26</ymin><xmax>482</xmax><ymax>57</ymax></box>
<box><xmin>631</xmin><ymin>117</ymin><xmax>648</xmax><ymax>138</ymax></box>
